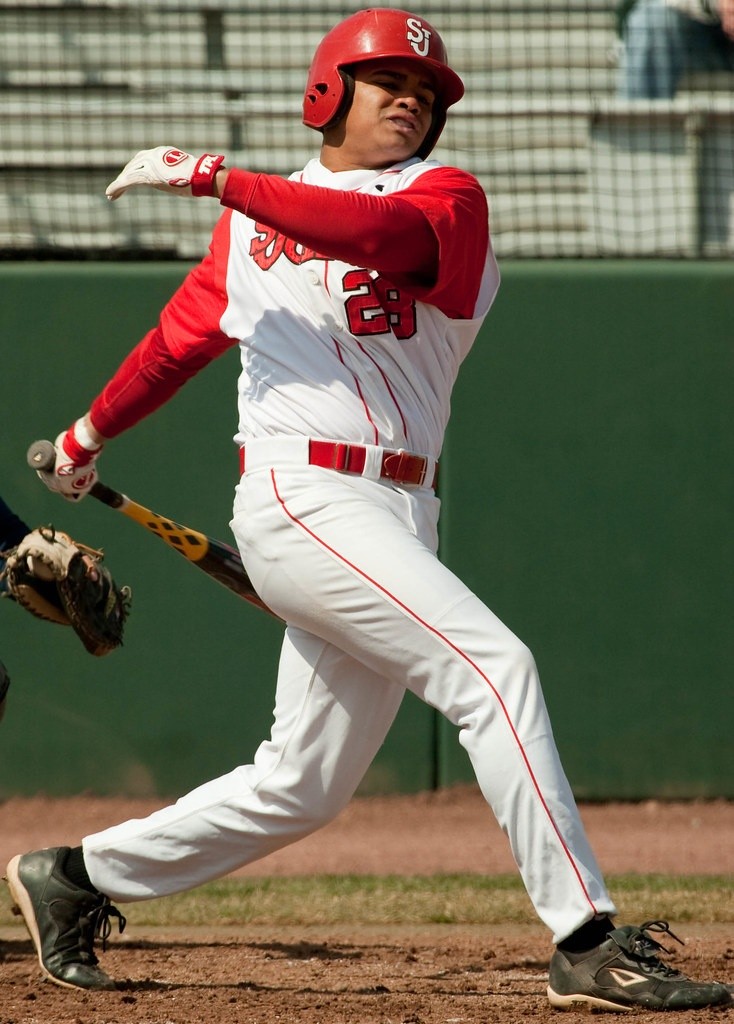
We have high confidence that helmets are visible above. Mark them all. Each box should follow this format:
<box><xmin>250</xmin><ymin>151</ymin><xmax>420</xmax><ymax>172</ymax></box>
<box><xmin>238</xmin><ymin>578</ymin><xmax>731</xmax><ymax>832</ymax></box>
<box><xmin>302</xmin><ymin>6</ymin><xmax>465</xmax><ymax>160</ymax></box>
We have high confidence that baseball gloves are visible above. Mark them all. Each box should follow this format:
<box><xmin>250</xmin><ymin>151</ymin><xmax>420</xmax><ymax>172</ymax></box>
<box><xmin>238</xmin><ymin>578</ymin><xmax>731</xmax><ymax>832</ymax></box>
<box><xmin>2</xmin><ymin>527</ymin><xmax>127</xmax><ymax>658</ymax></box>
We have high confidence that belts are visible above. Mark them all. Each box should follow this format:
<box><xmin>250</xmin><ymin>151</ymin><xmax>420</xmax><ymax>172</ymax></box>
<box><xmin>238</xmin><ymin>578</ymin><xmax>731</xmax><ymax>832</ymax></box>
<box><xmin>240</xmin><ymin>437</ymin><xmax>440</xmax><ymax>491</ymax></box>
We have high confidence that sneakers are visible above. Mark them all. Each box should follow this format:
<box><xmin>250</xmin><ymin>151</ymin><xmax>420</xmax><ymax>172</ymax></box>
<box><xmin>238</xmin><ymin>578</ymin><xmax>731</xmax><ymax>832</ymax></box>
<box><xmin>5</xmin><ymin>846</ymin><xmax>126</xmax><ymax>992</ymax></box>
<box><xmin>545</xmin><ymin>917</ymin><xmax>733</xmax><ymax>1012</ymax></box>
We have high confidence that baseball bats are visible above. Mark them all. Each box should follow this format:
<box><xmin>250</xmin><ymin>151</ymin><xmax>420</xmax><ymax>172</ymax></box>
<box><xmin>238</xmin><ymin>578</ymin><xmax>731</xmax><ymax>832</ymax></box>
<box><xmin>25</xmin><ymin>439</ymin><xmax>288</xmax><ymax>624</ymax></box>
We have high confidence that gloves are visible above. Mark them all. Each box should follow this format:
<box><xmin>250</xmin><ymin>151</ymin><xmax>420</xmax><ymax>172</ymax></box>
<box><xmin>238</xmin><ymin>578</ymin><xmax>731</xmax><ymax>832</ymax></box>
<box><xmin>33</xmin><ymin>418</ymin><xmax>104</xmax><ymax>504</ymax></box>
<box><xmin>104</xmin><ymin>144</ymin><xmax>226</xmax><ymax>201</ymax></box>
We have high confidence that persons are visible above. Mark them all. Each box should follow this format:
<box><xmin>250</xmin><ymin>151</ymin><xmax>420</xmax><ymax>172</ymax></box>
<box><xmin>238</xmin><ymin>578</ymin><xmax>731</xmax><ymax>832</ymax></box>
<box><xmin>0</xmin><ymin>494</ymin><xmax>133</xmax><ymax>657</ymax></box>
<box><xmin>5</xmin><ymin>8</ymin><xmax>734</xmax><ymax>1015</ymax></box>
<box><xmin>615</xmin><ymin>0</ymin><xmax>734</xmax><ymax>102</ymax></box>
